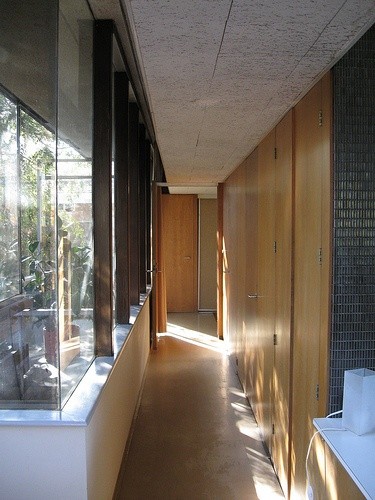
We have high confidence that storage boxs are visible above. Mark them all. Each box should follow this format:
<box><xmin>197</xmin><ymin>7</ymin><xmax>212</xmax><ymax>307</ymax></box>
<box><xmin>342</xmin><ymin>368</ymin><xmax>375</xmax><ymax>436</ymax></box>
<box><xmin>19</xmin><ymin>214</ymin><xmax>92</xmax><ymax>370</ymax></box>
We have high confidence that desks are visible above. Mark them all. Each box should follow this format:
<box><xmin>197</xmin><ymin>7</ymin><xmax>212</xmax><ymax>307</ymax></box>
<box><xmin>312</xmin><ymin>416</ymin><xmax>374</xmax><ymax>500</ymax></box>
